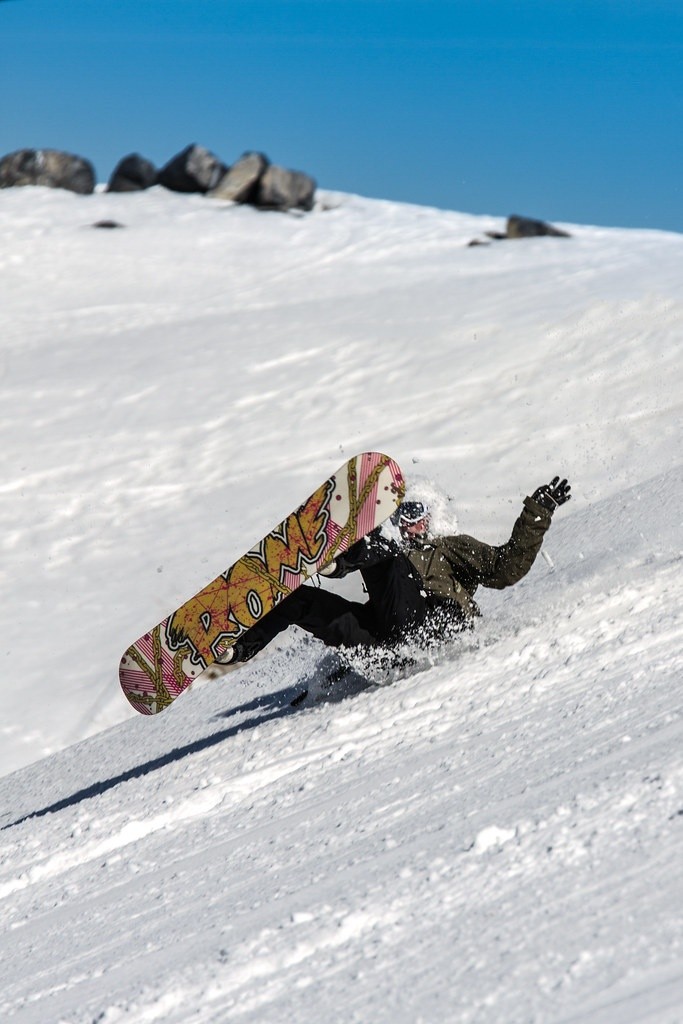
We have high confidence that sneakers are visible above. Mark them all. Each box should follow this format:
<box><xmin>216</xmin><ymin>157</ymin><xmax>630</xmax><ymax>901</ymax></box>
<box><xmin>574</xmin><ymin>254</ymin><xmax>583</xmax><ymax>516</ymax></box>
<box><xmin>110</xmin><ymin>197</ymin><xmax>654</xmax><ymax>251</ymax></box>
<box><xmin>319</xmin><ymin>560</ymin><xmax>363</xmax><ymax>580</ymax></box>
<box><xmin>213</xmin><ymin>643</ymin><xmax>244</xmax><ymax>666</ymax></box>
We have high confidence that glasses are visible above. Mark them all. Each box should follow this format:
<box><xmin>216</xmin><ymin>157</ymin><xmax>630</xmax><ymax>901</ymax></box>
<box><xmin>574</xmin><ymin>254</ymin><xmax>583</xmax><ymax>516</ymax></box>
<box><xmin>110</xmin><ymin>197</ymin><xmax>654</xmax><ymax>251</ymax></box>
<box><xmin>390</xmin><ymin>501</ymin><xmax>431</xmax><ymax>526</ymax></box>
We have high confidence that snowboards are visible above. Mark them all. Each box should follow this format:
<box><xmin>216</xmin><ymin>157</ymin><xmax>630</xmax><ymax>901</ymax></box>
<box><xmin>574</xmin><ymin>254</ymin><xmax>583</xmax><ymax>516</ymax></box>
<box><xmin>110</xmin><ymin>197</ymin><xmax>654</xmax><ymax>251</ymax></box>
<box><xmin>119</xmin><ymin>451</ymin><xmax>409</xmax><ymax>717</ymax></box>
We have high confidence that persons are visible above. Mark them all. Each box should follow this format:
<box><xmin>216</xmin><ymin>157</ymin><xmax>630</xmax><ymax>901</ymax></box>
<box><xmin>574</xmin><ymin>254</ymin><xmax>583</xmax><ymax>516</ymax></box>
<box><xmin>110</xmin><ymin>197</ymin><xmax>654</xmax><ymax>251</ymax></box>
<box><xmin>212</xmin><ymin>476</ymin><xmax>573</xmax><ymax>679</ymax></box>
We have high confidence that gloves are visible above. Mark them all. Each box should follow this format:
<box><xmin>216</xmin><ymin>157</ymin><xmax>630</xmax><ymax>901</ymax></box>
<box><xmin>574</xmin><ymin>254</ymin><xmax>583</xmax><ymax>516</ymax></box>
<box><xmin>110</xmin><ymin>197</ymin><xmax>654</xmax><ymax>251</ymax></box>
<box><xmin>531</xmin><ymin>475</ymin><xmax>573</xmax><ymax>511</ymax></box>
